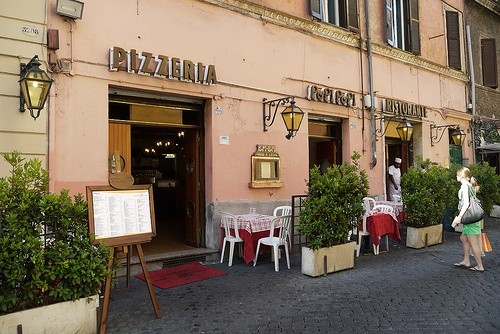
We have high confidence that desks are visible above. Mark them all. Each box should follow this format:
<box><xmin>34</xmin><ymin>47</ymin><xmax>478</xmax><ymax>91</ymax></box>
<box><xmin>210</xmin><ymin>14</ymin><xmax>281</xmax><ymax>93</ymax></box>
<box><xmin>369</xmin><ymin>201</ymin><xmax>407</xmax><ymax>222</ymax></box>
<box><xmin>358</xmin><ymin>211</ymin><xmax>401</xmax><ymax>255</ymax></box>
<box><xmin>220</xmin><ymin>214</ymin><xmax>290</xmax><ymax>265</ymax></box>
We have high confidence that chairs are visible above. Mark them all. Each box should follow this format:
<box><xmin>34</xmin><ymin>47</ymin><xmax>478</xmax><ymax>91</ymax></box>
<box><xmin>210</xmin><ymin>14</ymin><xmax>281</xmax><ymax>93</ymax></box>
<box><xmin>273</xmin><ymin>206</ymin><xmax>291</xmax><ymax>248</ymax></box>
<box><xmin>363</xmin><ymin>197</ymin><xmax>393</xmax><ymax>251</ymax></box>
<box><xmin>253</xmin><ymin>215</ymin><xmax>291</xmax><ymax>272</ymax></box>
<box><xmin>219</xmin><ymin>211</ymin><xmax>243</xmax><ymax>266</ymax></box>
<box><xmin>347</xmin><ymin>211</ymin><xmax>376</xmax><ymax>257</ymax></box>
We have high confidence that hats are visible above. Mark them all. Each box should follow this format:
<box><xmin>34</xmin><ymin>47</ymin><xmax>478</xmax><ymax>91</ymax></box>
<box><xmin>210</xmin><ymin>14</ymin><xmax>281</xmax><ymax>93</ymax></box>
<box><xmin>395</xmin><ymin>158</ymin><xmax>402</xmax><ymax>163</ymax></box>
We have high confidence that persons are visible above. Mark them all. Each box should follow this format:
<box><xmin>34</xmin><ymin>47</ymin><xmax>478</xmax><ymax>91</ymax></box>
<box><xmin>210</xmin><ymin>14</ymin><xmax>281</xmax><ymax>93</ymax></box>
<box><xmin>387</xmin><ymin>158</ymin><xmax>402</xmax><ymax>202</ymax></box>
<box><xmin>452</xmin><ymin>167</ymin><xmax>485</xmax><ymax>272</ymax></box>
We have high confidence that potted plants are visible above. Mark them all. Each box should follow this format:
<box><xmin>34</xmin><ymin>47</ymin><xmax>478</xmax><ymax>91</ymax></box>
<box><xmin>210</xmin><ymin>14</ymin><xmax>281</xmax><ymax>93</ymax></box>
<box><xmin>0</xmin><ymin>150</ymin><xmax>123</xmax><ymax>334</ymax></box>
<box><xmin>455</xmin><ymin>162</ymin><xmax>500</xmax><ymax>232</ymax></box>
<box><xmin>295</xmin><ymin>151</ymin><xmax>371</xmax><ymax>277</ymax></box>
<box><xmin>399</xmin><ymin>155</ymin><xmax>449</xmax><ymax>248</ymax></box>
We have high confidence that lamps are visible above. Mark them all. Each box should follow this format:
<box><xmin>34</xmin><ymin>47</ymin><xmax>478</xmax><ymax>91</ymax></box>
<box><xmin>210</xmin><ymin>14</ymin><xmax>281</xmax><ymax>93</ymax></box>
<box><xmin>262</xmin><ymin>97</ymin><xmax>304</xmax><ymax>140</ymax></box>
<box><xmin>430</xmin><ymin>124</ymin><xmax>466</xmax><ymax>147</ymax></box>
<box><xmin>18</xmin><ymin>55</ymin><xmax>54</xmax><ymax>121</ymax></box>
<box><xmin>375</xmin><ymin>112</ymin><xmax>414</xmax><ymax>142</ymax></box>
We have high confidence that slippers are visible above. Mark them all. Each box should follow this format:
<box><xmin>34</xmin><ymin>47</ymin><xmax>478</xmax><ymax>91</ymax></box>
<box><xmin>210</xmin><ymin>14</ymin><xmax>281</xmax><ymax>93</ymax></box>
<box><xmin>453</xmin><ymin>261</ymin><xmax>470</xmax><ymax>268</ymax></box>
<box><xmin>469</xmin><ymin>266</ymin><xmax>484</xmax><ymax>272</ymax></box>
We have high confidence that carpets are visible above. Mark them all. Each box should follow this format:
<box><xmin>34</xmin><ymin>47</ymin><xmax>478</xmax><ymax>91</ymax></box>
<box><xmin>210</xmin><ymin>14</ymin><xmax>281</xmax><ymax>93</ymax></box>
<box><xmin>134</xmin><ymin>263</ymin><xmax>227</xmax><ymax>289</ymax></box>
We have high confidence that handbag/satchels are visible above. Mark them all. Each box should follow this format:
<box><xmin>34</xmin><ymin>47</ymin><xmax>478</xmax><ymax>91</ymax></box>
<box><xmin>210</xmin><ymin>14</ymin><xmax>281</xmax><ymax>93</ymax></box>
<box><xmin>469</xmin><ymin>232</ymin><xmax>492</xmax><ymax>256</ymax></box>
<box><xmin>458</xmin><ymin>186</ymin><xmax>485</xmax><ymax>224</ymax></box>
